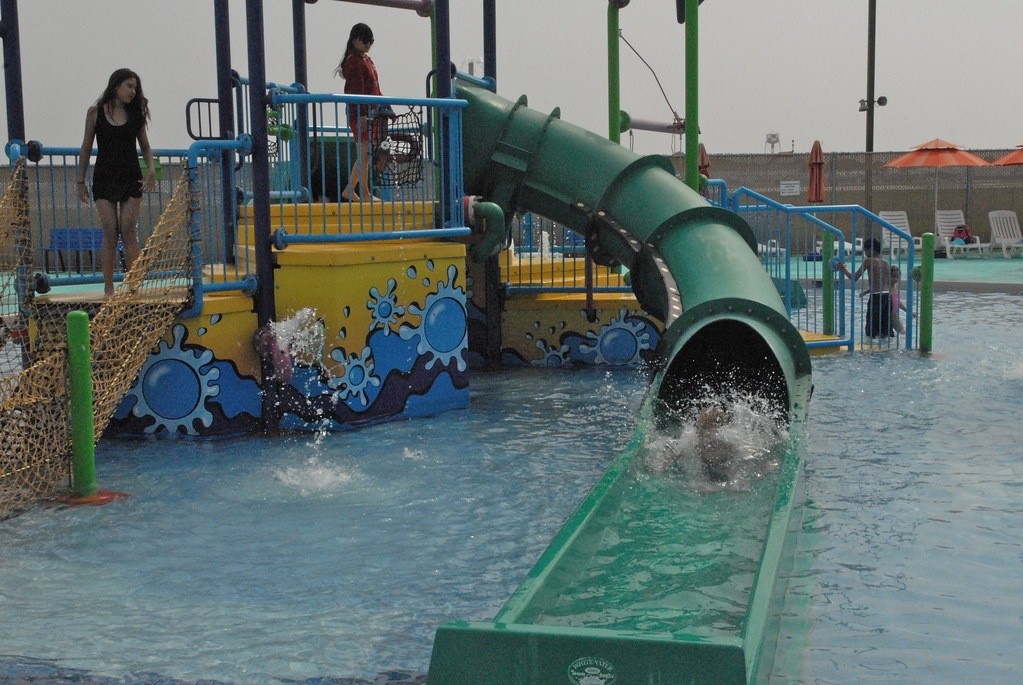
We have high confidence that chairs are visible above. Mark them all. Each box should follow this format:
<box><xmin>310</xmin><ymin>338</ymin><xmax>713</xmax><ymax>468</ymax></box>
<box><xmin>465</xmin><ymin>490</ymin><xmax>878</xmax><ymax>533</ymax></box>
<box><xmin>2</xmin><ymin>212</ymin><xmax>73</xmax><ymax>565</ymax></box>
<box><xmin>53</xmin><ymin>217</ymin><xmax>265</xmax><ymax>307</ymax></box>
<box><xmin>878</xmin><ymin>209</ymin><xmax>923</xmax><ymax>256</ymax></box>
<box><xmin>756</xmin><ymin>239</ymin><xmax>785</xmax><ymax>260</ymax></box>
<box><xmin>934</xmin><ymin>209</ymin><xmax>989</xmax><ymax>260</ymax></box>
<box><xmin>986</xmin><ymin>209</ymin><xmax>1023</xmax><ymax>258</ymax></box>
<box><xmin>816</xmin><ymin>235</ymin><xmax>863</xmax><ymax>255</ymax></box>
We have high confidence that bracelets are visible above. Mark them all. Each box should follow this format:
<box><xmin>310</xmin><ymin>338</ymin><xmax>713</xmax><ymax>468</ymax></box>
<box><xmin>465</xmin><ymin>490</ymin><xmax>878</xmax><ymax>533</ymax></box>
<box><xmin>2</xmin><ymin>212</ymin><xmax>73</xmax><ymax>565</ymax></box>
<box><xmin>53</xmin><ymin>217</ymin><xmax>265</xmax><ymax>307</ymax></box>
<box><xmin>77</xmin><ymin>182</ymin><xmax>85</xmax><ymax>184</ymax></box>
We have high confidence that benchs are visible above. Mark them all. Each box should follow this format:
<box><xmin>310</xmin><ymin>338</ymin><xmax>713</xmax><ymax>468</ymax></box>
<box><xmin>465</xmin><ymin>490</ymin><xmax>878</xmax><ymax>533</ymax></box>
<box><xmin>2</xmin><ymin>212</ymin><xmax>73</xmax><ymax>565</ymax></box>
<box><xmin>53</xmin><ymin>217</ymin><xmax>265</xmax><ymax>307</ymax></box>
<box><xmin>34</xmin><ymin>227</ymin><xmax>130</xmax><ymax>273</ymax></box>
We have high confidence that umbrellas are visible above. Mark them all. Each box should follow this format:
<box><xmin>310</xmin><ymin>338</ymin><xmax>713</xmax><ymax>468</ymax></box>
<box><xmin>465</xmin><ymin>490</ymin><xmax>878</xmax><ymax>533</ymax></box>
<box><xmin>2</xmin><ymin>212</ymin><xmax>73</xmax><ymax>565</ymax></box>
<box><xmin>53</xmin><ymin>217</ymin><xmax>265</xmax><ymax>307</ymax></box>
<box><xmin>883</xmin><ymin>138</ymin><xmax>993</xmax><ymax>249</ymax></box>
<box><xmin>698</xmin><ymin>143</ymin><xmax>712</xmax><ymax>199</ymax></box>
<box><xmin>808</xmin><ymin>141</ymin><xmax>824</xmax><ymax>254</ymax></box>
<box><xmin>993</xmin><ymin>145</ymin><xmax>1023</xmax><ymax>166</ymax></box>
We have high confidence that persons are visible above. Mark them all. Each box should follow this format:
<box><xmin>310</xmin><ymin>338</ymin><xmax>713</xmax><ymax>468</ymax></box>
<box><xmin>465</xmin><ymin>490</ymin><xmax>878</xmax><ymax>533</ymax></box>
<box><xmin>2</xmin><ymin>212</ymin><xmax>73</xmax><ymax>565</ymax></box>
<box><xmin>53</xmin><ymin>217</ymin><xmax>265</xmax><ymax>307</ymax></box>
<box><xmin>838</xmin><ymin>239</ymin><xmax>919</xmax><ymax>340</ymax></box>
<box><xmin>77</xmin><ymin>69</ymin><xmax>156</xmax><ymax>301</ymax></box>
<box><xmin>341</xmin><ymin>23</ymin><xmax>397</xmax><ymax>202</ymax></box>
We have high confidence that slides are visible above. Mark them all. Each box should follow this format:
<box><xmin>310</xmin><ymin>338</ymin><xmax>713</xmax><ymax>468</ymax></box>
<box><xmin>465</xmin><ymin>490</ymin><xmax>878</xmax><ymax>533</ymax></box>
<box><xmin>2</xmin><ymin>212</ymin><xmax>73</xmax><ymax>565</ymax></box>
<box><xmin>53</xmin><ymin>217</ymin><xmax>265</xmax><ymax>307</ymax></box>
<box><xmin>426</xmin><ymin>80</ymin><xmax>815</xmax><ymax>685</ymax></box>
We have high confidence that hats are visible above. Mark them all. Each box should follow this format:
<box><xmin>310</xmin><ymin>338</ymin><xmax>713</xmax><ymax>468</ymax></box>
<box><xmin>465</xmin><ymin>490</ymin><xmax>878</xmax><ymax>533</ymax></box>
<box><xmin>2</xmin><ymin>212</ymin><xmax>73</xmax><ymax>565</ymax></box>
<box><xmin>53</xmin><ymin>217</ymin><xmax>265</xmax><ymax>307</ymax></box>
<box><xmin>863</xmin><ymin>237</ymin><xmax>880</xmax><ymax>251</ymax></box>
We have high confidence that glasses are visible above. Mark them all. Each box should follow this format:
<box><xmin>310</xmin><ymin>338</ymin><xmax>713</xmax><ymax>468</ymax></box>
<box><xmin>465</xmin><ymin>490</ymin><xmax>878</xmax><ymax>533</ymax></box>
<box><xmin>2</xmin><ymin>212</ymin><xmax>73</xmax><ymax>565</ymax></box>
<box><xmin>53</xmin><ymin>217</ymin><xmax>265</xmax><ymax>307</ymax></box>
<box><xmin>359</xmin><ymin>37</ymin><xmax>375</xmax><ymax>45</ymax></box>
